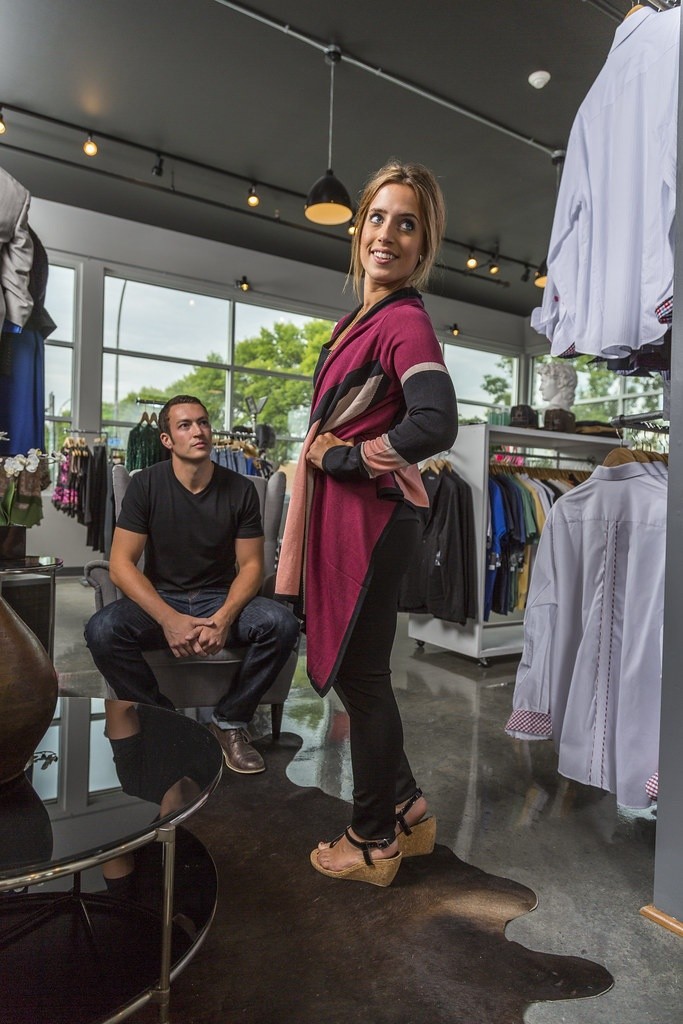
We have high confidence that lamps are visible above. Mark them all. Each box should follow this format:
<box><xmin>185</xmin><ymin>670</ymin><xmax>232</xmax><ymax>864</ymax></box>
<box><xmin>83</xmin><ymin>132</ymin><xmax>98</xmax><ymax>157</ymax></box>
<box><xmin>450</xmin><ymin>323</ymin><xmax>460</xmax><ymax>336</ymax></box>
<box><xmin>304</xmin><ymin>43</ymin><xmax>353</xmax><ymax>227</ymax></box>
<box><xmin>534</xmin><ymin>256</ymin><xmax>548</xmax><ymax>289</ymax></box>
<box><xmin>247</xmin><ymin>181</ymin><xmax>260</xmax><ymax>208</ymax></box>
<box><xmin>152</xmin><ymin>152</ymin><xmax>165</xmax><ymax>177</ymax></box>
<box><xmin>466</xmin><ymin>245</ymin><xmax>500</xmax><ymax>274</ymax></box>
<box><xmin>235</xmin><ymin>275</ymin><xmax>250</xmax><ymax>291</ymax></box>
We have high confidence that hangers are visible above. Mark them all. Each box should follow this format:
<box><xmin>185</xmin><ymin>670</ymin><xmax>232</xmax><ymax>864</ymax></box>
<box><xmin>622</xmin><ymin>0</ymin><xmax>643</xmax><ymax>21</ymax></box>
<box><xmin>137</xmin><ymin>399</ymin><xmax>151</xmax><ymax>426</ymax></box>
<box><xmin>420</xmin><ymin>450</ymin><xmax>453</xmax><ymax>476</ymax></box>
<box><xmin>485</xmin><ymin>449</ymin><xmax>593</xmax><ymax>484</ymax></box>
<box><xmin>599</xmin><ymin>417</ymin><xmax>670</xmax><ymax>468</ymax></box>
<box><xmin>62</xmin><ymin>429</ymin><xmax>105</xmax><ymax>457</ymax></box>
<box><xmin>148</xmin><ymin>400</ymin><xmax>159</xmax><ymax>427</ymax></box>
<box><xmin>211</xmin><ymin>428</ymin><xmax>261</xmax><ymax>459</ymax></box>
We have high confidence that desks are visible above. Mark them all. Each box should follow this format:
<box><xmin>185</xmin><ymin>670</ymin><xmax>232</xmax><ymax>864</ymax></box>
<box><xmin>0</xmin><ymin>697</ymin><xmax>223</xmax><ymax>1024</ymax></box>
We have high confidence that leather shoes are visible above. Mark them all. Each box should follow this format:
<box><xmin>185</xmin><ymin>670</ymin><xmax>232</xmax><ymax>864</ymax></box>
<box><xmin>210</xmin><ymin>718</ymin><xmax>266</xmax><ymax>773</ymax></box>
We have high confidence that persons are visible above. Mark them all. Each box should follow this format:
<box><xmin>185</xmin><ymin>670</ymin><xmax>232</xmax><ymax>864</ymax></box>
<box><xmin>0</xmin><ymin>164</ymin><xmax>52</xmax><ymax>458</ymax></box>
<box><xmin>83</xmin><ymin>396</ymin><xmax>298</xmax><ymax>774</ymax></box>
<box><xmin>538</xmin><ymin>362</ymin><xmax>577</xmax><ymax>413</ymax></box>
<box><xmin>272</xmin><ymin>160</ymin><xmax>456</xmax><ymax>890</ymax></box>
<box><xmin>102</xmin><ymin>698</ymin><xmax>221</xmax><ymax>824</ymax></box>
<box><xmin>100</xmin><ymin>823</ymin><xmax>217</xmax><ymax>971</ymax></box>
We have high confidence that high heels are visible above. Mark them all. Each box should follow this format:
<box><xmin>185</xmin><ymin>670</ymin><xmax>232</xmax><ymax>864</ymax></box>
<box><xmin>310</xmin><ymin>823</ymin><xmax>404</xmax><ymax>887</ymax></box>
<box><xmin>395</xmin><ymin>787</ymin><xmax>437</xmax><ymax>855</ymax></box>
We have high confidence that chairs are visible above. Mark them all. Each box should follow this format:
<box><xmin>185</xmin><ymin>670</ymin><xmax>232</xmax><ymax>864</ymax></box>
<box><xmin>83</xmin><ymin>461</ymin><xmax>303</xmax><ymax>742</ymax></box>
